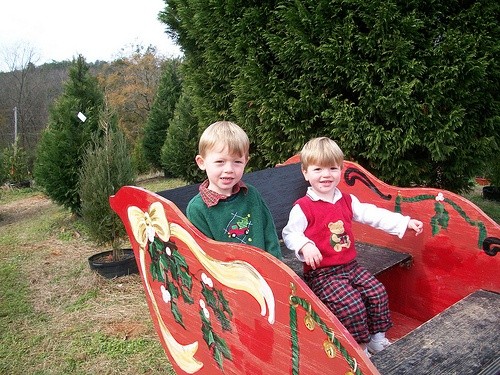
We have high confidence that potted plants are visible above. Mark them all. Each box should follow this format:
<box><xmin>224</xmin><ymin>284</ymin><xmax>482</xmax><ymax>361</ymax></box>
<box><xmin>88</xmin><ymin>104</ymin><xmax>140</xmax><ymax>279</ymax></box>
<box><xmin>469</xmin><ymin>134</ymin><xmax>499</xmax><ymax>186</ymax></box>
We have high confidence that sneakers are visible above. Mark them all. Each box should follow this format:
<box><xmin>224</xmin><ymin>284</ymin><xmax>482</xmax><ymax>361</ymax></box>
<box><xmin>367</xmin><ymin>338</ymin><xmax>393</xmax><ymax>355</ymax></box>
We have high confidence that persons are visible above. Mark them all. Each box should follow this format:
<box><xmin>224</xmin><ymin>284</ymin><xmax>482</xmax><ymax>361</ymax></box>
<box><xmin>184</xmin><ymin>122</ymin><xmax>281</xmax><ymax>261</ymax></box>
<box><xmin>281</xmin><ymin>135</ymin><xmax>423</xmax><ymax>359</ymax></box>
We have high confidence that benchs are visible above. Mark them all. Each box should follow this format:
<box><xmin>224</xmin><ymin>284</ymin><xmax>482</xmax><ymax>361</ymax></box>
<box><xmin>152</xmin><ymin>161</ymin><xmax>412</xmax><ymax>304</ymax></box>
<box><xmin>369</xmin><ymin>289</ymin><xmax>500</xmax><ymax>375</ymax></box>
